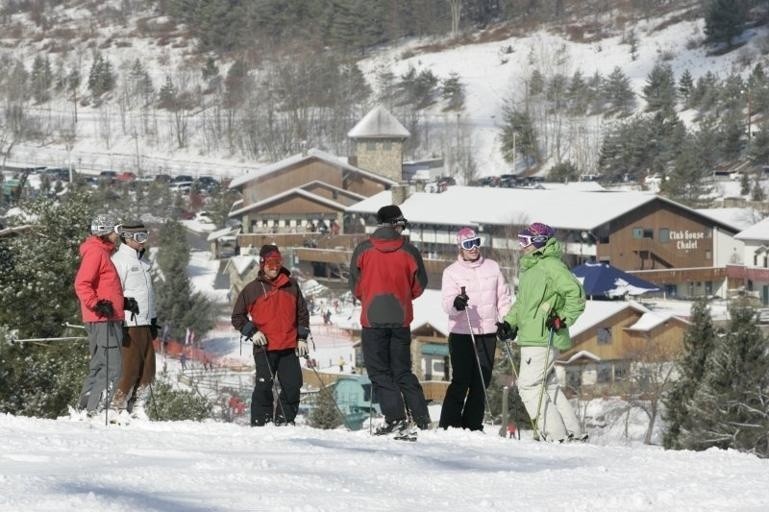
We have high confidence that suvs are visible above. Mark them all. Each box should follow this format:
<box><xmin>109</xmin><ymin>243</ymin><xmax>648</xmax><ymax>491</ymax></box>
<box><xmin>228</xmin><ymin>396</ymin><xmax>250</xmax><ymax>415</ymax></box>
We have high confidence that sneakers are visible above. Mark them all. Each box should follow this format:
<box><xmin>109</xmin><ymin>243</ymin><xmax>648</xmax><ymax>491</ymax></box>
<box><xmin>74</xmin><ymin>408</ymin><xmax>148</xmax><ymax>425</ymax></box>
<box><xmin>395</xmin><ymin>425</ymin><xmax>418</xmax><ymax>441</ymax></box>
<box><xmin>376</xmin><ymin>418</ymin><xmax>408</xmax><ymax>435</ymax></box>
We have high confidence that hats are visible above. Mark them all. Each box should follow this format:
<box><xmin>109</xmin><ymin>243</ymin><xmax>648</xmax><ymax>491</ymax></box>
<box><xmin>523</xmin><ymin>223</ymin><xmax>553</xmax><ymax>248</ymax></box>
<box><xmin>457</xmin><ymin>227</ymin><xmax>476</xmax><ymax>241</ymax></box>
<box><xmin>91</xmin><ymin>214</ymin><xmax>119</xmax><ymax>236</ymax></box>
<box><xmin>119</xmin><ymin>220</ymin><xmax>147</xmax><ymax>232</ymax></box>
<box><xmin>260</xmin><ymin>245</ymin><xmax>281</xmax><ymax>256</ymax></box>
<box><xmin>377</xmin><ymin>205</ymin><xmax>402</xmax><ymax>221</ymax></box>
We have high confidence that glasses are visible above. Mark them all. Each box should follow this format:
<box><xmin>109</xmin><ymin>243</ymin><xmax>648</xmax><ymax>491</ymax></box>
<box><xmin>397</xmin><ymin>217</ymin><xmax>407</xmax><ymax>229</ymax></box>
<box><xmin>265</xmin><ymin>258</ymin><xmax>281</xmax><ymax>267</ymax></box>
<box><xmin>122</xmin><ymin>230</ymin><xmax>150</xmax><ymax>243</ymax></box>
<box><xmin>517</xmin><ymin>234</ymin><xmax>548</xmax><ymax>248</ymax></box>
<box><xmin>91</xmin><ymin>225</ymin><xmax>122</xmax><ymax>235</ymax></box>
<box><xmin>462</xmin><ymin>237</ymin><xmax>480</xmax><ymax>250</ymax></box>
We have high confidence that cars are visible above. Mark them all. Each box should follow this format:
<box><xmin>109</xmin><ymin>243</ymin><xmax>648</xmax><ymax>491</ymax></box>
<box><xmin>297</xmin><ymin>394</ymin><xmax>320</xmax><ymax>415</ymax></box>
<box><xmin>729</xmin><ymin>169</ymin><xmax>758</xmax><ymax>182</ymax></box>
<box><xmin>467</xmin><ymin>173</ymin><xmax>545</xmax><ymax>187</ymax></box>
<box><xmin>38</xmin><ymin>166</ymin><xmax>223</xmax><ymax>200</ymax></box>
<box><xmin>643</xmin><ymin>174</ymin><xmax>663</xmax><ymax>184</ymax></box>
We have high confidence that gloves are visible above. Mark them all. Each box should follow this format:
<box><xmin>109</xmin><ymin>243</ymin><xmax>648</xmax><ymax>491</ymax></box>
<box><xmin>96</xmin><ymin>299</ymin><xmax>112</xmax><ymax>317</ymax></box>
<box><xmin>294</xmin><ymin>339</ymin><xmax>309</xmax><ymax>357</ymax></box>
<box><xmin>250</xmin><ymin>330</ymin><xmax>267</xmax><ymax>346</ymax></box>
<box><xmin>546</xmin><ymin>315</ymin><xmax>566</xmax><ymax>331</ymax></box>
<box><xmin>496</xmin><ymin>322</ymin><xmax>518</xmax><ymax>341</ymax></box>
<box><xmin>454</xmin><ymin>294</ymin><xmax>469</xmax><ymax>310</ymax></box>
<box><xmin>124</xmin><ymin>297</ymin><xmax>139</xmax><ymax>315</ymax></box>
<box><xmin>151</xmin><ymin>318</ymin><xmax>161</xmax><ymax>339</ymax></box>
<box><xmin>122</xmin><ymin>327</ymin><xmax>131</xmax><ymax>347</ymax></box>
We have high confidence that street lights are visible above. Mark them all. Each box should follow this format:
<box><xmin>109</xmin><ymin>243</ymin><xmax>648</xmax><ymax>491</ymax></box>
<box><xmin>512</xmin><ymin>127</ymin><xmax>523</xmax><ymax>172</ymax></box>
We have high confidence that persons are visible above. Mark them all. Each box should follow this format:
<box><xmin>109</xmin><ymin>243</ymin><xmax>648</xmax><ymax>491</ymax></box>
<box><xmin>348</xmin><ymin>205</ymin><xmax>431</xmax><ymax>437</ymax></box>
<box><xmin>309</xmin><ymin>296</ymin><xmax>356</xmax><ymax>326</ymax></box>
<box><xmin>232</xmin><ymin>245</ymin><xmax>310</xmax><ymax>428</ymax></box>
<box><xmin>439</xmin><ymin>227</ymin><xmax>514</xmax><ymax>432</ymax></box>
<box><xmin>180</xmin><ymin>352</ymin><xmax>187</xmax><ymax>371</ymax></box>
<box><xmin>73</xmin><ymin>213</ymin><xmax>125</xmax><ymax>418</ymax></box>
<box><xmin>110</xmin><ymin>220</ymin><xmax>162</xmax><ymax>426</ymax></box>
<box><xmin>200</xmin><ymin>354</ymin><xmax>209</xmax><ymax>369</ymax></box>
<box><xmin>316</xmin><ymin>220</ymin><xmax>327</xmax><ymax>233</ymax></box>
<box><xmin>329</xmin><ymin>219</ymin><xmax>339</xmax><ymax>236</ymax></box>
<box><xmin>311</xmin><ymin>224</ymin><xmax>316</xmax><ymax>233</ymax></box>
<box><xmin>493</xmin><ymin>222</ymin><xmax>588</xmax><ymax>445</ymax></box>
